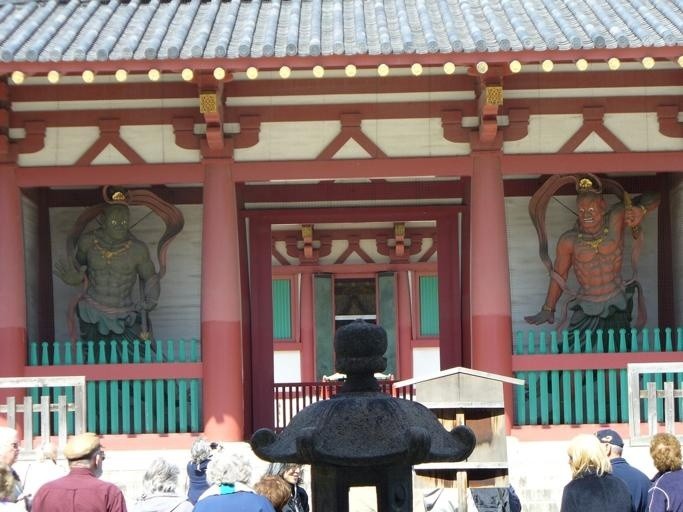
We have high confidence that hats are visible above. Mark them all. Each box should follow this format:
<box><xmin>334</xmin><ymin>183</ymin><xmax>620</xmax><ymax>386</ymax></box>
<box><xmin>594</xmin><ymin>428</ymin><xmax>624</xmax><ymax>449</ymax></box>
<box><xmin>63</xmin><ymin>432</ymin><xmax>102</xmax><ymax>462</ymax></box>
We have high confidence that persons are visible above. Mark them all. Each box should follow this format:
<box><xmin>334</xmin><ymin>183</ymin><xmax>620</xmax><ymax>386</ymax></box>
<box><xmin>421</xmin><ymin>425</ymin><xmax>683</xmax><ymax>512</ymax></box>
<box><xmin>51</xmin><ymin>187</ymin><xmax>187</xmax><ymax>408</ymax></box>
<box><xmin>523</xmin><ymin>174</ymin><xmax>661</xmax><ymax>395</ymax></box>
<box><xmin>1</xmin><ymin>425</ymin><xmax>310</xmax><ymax>511</ymax></box>
